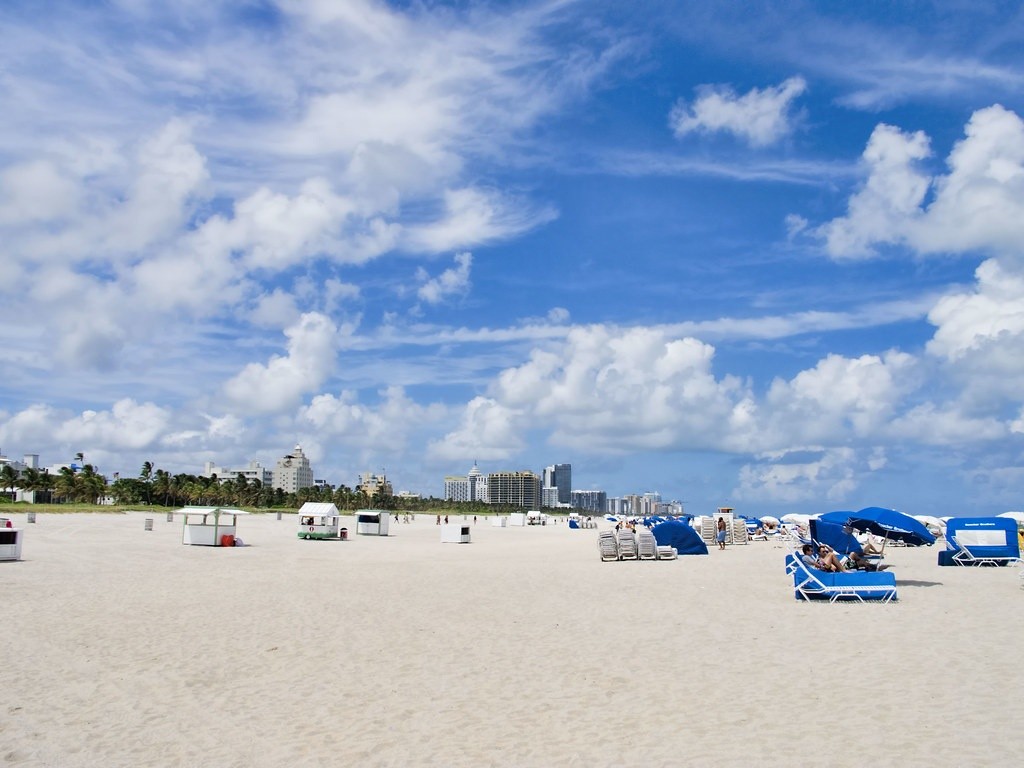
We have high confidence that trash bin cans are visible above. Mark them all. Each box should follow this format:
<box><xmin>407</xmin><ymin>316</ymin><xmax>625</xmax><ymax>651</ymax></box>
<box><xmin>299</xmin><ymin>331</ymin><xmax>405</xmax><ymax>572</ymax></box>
<box><xmin>145</xmin><ymin>519</ymin><xmax>153</xmax><ymax>531</ymax></box>
<box><xmin>27</xmin><ymin>511</ymin><xmax>36</xmax><ymax>523</ymax></box>
<box><xmin>277</xmin><ymin>511</ymin><xmax>282</xmax><ymax>520</ymax></box>
<box><xmin>340</xmin><ymin>528</ymin><xmax>348</xmax><ymax>541</ymax></box>
<box><xmin>167</xmin><ymin>512</ymin><xmax>173</xmax><ymax>522</ymax></box>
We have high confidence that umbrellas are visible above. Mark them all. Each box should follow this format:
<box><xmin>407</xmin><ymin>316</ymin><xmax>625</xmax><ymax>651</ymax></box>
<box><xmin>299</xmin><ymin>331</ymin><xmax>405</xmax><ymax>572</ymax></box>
<box><xmin>995</xmin><ymin>511</ymin><xmax>1024</xmax><ymax>524</ymax></box>
<box><xmin>780</xmin><ymin>513</ymin><xmax>822</xmax><ymax>526</ymax></box>
<box><xmin>913</xmin><ymin>516</ymin><xmax>954</xmax><ymax>527</ymax></box>
<box><xmin>817</xmin><ymin>510</ymin><xmax>857</xmax><ymax>525</ymax></box>
<box><xmin>845</xmin><ymin>507</ymin><xmax>936</xmax><ymax>571</ymax></box>
<box><xmin>759</xmin><ymin>516</ymin><xmax>780</xmax><ymax>526</ymax></box>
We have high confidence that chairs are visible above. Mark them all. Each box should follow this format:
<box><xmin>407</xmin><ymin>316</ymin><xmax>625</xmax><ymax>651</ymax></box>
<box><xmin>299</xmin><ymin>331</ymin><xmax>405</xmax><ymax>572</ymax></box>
<box><xmin>580</xmin><ymin>516</ymin><xmax>1024</xmax><ymax>605</ymax></box>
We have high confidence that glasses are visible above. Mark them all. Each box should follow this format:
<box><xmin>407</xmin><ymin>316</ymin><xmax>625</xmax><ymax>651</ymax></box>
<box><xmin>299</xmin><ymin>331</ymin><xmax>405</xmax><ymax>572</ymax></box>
<box><xmin>809</xmin><ymin>549</ymin><xmax>813</xmax><ymax>552</ymax></box>
<box><xmin>822</xmin><ymin>551</ymin><xmax>828</xmax><ymax>553</ymax></box>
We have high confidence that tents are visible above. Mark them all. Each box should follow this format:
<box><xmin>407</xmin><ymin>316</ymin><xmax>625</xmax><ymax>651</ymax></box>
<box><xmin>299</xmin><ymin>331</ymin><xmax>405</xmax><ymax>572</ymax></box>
<box><xmin>809</xmin><ymin>519</ymin><xmax>862</xmax><ymax>558</ymax></box>
<box><xmin>738</xmin><ymin>515</ymin><xmax>762</xmax><ymax>535</ymax></box>
<box><xmin>637</xmin><ymin>514</ymin><xmax>708</xmax><ymax>555</ymax></box>
<box><xmin>569</xmin><ymin>519</ymin><xmax>578</xmax><ymax>528</ymax></box>
<box><xmin>938</xmin><ymin>517</ymin><xmax>1020</xmax><ymax>567</ymax></box>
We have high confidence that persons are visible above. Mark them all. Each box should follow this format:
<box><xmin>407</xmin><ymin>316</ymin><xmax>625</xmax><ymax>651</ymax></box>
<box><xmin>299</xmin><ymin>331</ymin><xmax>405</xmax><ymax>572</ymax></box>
<box><xmin>925</xmin><ymin>523</ymin><xmax>938</xmax><ymax>537</ymax></box>
<box><xmin>615</xmin><ymin>519</ymin><xmax>636</xmax><ymax>535</ymax></box>
<box><xmin>802</xmin><ymin>544</ymin><xmax>851</xmax><ymax>573</ymax></box>
<box><xmin>755</xmin><ymin>527</ymin><xmax>761</xmax><ymax>535</ymax></box>
<box><xmin>817</xmin><ymin>546</ymin><xmax>870</xmax><ymax>570</ymax></box>
<box><xmin>718</xmin><ymin>517</ymin><xmax>727</xmax><ymax>550</ymax></box>
<box><xmin>394</xmin><ymin>513</ymin><xmax>400</xmax><ymax>523</ymax></box>
<box><xmin>746</xmin><ymin>532</ymin><xmax>753</xmax><ymax>541</ymax></box>
<box><xmin>863</xmin><ymin>543</ymin><xmax>881</xmax><ymax>555</ymax></box>
<box><xmin>444</xmin><ymin>515</ymin><xmax>448</xmax><ymax>524</ymax></box>
<box><xmin>781</xmin><ymin>526</ymin><xmax>787</xmax><ymax>535</ymax></box>
<box><xmin>436</xmin><ymin>515</ymin><xmax>441</xmax><ymax>525</ymax></box>
